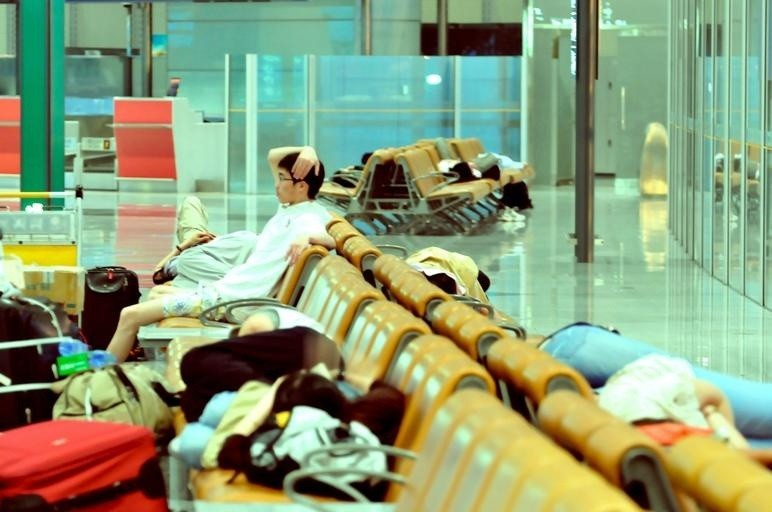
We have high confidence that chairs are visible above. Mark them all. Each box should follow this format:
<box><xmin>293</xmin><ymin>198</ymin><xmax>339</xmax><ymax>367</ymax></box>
<box><xmin>318</xmin><ymin>138</ymin><xmax>535</xmax><ymax>235</ymax></box>
<box><xmin>713</xmin><ymin>139</ymin><xmax>760</xmax><ymax>195</ymax></box>
<box><xmin>133</xmin><ymin>214</ymin><xmax>771</xmax><ymax>512</ymax></box>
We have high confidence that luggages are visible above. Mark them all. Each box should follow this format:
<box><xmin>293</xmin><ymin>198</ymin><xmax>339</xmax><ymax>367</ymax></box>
<box><xmin>83</xmin><ymin>265</ymin><xmax>141</xmax><ymax>351</ymax></box>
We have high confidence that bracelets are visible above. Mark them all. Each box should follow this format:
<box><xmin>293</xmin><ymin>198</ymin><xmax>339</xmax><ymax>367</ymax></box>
<box><xmin>176</xmin><ymin>245</ymin><xmax>182</xmax><ymax>252</ymax></box>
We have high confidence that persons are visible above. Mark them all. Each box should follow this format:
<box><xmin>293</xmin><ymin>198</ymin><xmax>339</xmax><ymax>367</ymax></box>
<box><xmin>153</xmin><ymin>197</ymin><xmax>258</xmax><ymax>291</ymax></box>
<box><xmin>411</xmin><ymin>247</ymin><xmax>490</xmax><ymax>316</ymax></box>
<box><xmin>597</xmin><ymin>354</ymin><xmax>772</xmax><ymax>470</ymax></box>
<box><xmin>106</xmin><ymin>147</ymin><xmax>336</xmax><ymax>364</ymax></box>
<box><xmin>181</xmin><ymin>306</ymin><xmax>344</xmax><ymax>423</ymax></box>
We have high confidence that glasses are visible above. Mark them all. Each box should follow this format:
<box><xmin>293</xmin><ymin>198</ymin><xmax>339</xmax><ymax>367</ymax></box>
<box><xmin>277</xmin><ymin>174</ymin><xmax>295</xmax><ymax>183</ymax></box>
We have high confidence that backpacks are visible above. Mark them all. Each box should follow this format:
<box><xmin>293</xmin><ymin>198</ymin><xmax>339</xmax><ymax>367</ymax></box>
<box><xmin>53</xmin><ymin>361</ymin><xmax>181</xmax><ymax>434</ymax></box>
